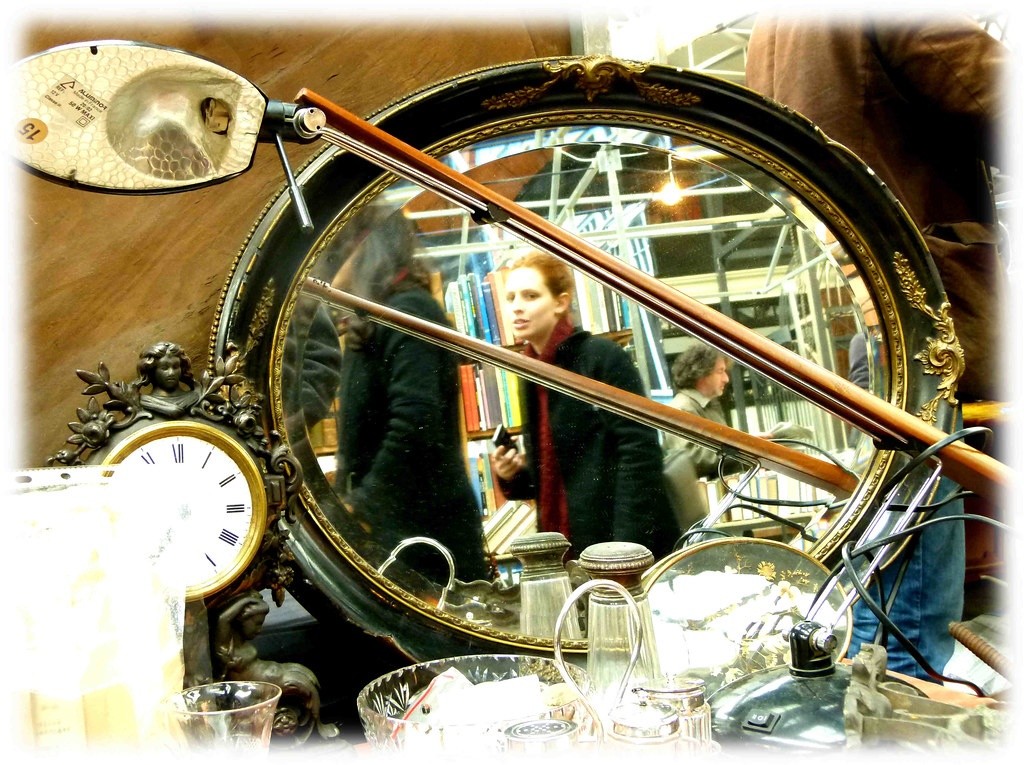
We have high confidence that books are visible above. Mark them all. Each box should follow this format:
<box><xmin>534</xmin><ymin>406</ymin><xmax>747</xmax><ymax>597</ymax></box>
<box><xmin>309</xmin><ymin>251</ymin><xmax>846</xmax><ymax>555</ymax></box>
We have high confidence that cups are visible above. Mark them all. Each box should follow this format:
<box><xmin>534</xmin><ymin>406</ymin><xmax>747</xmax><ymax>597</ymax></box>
<box><xmin>160</xmin><ymin>680</ymin><xmax>282</xmax><ymax>765</ymax></box>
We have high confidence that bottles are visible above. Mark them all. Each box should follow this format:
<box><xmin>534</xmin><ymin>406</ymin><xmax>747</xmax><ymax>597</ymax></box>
<box><xmin>606</xmin><ymin>701</ymin><xmax>681</xmax><ymax>765</ymax></box>
<box><xmin>579</xmin><ymin>542</ymin><xmax>660</xmax><ymax>724</ymax></box>
<box><xmin>510</xmin><ymin>532</ymin><xmax>582</xmax><ymax>641</ymax></box>
<box><xmin>504</xmin><ymin>719</ymin><xmax>580</xmax><ymax>754</ymax></box>
<box><xmin>639</xmin><ymin>676</ymin><xmax>713</xmax><ymax>765</ymax></box>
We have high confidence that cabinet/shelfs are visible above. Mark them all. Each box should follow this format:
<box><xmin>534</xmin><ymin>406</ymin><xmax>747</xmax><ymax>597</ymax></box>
<box><xmin>341</xmin><ymin>202</ymin><xmax>678</xmax><ymax>523</ymax></box>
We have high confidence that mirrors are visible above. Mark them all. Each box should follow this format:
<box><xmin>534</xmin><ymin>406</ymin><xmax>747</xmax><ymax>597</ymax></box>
<box><xmin>207</xmin><ymin>55</ymin><xmax>960</xmax><ymax>682</ymax></box>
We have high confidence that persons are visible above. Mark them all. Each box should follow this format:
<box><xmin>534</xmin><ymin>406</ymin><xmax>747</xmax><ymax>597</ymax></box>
<box><xmin>847</xmin><ymin>331</ymin><xmax>869</xmax><ymax>395</ymax></box>
<box><xmin>299</xmin><ymin>303</ymin><xmax>341</xmax><ymax>429</ymax></box>
<box><xmin>331</xmin><ymin>208</ymin><xmax>487</xmax><ymax>587</ymax></box>
<box><xmin>661</xmin><ymin>344</ymin><xmax>771</xmax><ymax>479</ymax></box>
<box><xmin>492</xmin><ymin>253</ymin><xmax>675</xmax><ymax>573</ymax></box>
<box><xmin>743</xmin><ymin>19</ymin><xmax>1004</xmax><ymax>684</ymax></box>
<box><xmin>136</xmin><ymin>340</ymin><xmax>197</xmax><ymax>398</ymax></box>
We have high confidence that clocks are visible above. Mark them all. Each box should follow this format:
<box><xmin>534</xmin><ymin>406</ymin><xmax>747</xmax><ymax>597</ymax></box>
<box><xmin>57</xmin><ymin>334</ymin><xmax>319</xmax><ymax>745</ymax></box>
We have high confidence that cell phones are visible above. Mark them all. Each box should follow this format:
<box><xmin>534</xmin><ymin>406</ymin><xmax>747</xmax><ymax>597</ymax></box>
<box><xmin>492</xmin><ymin>424</ymin><xmax>519</xmax><ymax>456</ymax></box>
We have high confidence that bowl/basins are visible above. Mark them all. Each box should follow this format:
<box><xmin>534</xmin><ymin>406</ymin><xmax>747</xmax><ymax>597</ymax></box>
<box><xmin>356</xmin><ymin>654</ymin><xmax>588</xmax><ymax>752</ymax></box>
